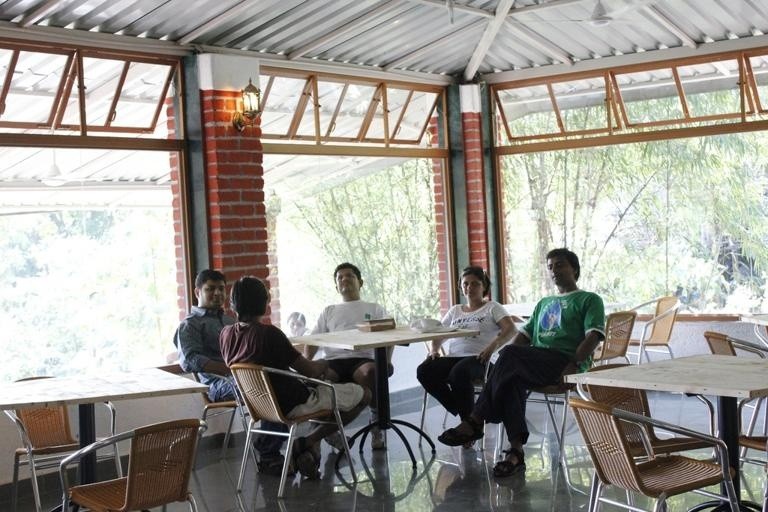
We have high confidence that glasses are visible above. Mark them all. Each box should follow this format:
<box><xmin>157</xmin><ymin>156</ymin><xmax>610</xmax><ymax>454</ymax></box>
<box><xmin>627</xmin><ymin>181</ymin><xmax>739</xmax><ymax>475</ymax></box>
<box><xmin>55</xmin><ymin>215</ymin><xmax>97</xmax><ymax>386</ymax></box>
<box><xmin>464</xmin><ymin>265</ymin><xmax>485</xmax><ymax>283</ymax></box>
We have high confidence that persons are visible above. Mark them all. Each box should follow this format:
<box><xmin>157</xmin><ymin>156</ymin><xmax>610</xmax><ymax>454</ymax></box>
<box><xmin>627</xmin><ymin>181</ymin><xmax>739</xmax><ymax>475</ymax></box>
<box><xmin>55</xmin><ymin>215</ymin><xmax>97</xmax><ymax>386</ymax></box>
<box><xmin>287</xmin><ymin>312</ymin><xmax>311</xmax><ymax>337</ymax></box>
<box><xmin>303</xmin><ymin>262</ymin><xmax>395</xmax><ymax>452</ymax></box>
<box><xmin>218</xmin><ymin>276</ymin><xmax>373</xmax><ymax>480</ymax></box>
<box><xmin>416</xmin><ymin>266</ymin><xmax>518</xmax><ymax>449</ymax></box>
<box><xmin>438</xmin><ymin>248</ymin><xmax>606</xmax><ymax>478</ymax></box>
<box><xmin>173</xmin><ymin>270</ymin><xmax>292</xmax><ymax>475</ymax></box>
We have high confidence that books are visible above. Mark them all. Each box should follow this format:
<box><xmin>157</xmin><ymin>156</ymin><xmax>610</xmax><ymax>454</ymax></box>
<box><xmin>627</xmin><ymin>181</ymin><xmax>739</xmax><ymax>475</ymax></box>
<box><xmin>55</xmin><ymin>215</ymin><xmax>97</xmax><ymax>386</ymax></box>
<box><xmin>357</xmin><ymin>319</ymin><xmax>395</xmax><ymax>332</ymax></box>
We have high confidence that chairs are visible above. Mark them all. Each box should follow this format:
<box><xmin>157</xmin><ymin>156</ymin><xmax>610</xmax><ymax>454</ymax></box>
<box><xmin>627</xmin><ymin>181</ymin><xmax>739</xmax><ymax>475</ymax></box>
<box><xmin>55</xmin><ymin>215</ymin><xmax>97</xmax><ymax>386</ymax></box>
<box><xmin>190</xmin><ymin>370</ymin><xmax>260</xmax><ymax>474</ymax></box>
<box><xmin>628</xmin><ymin>296</ymin><xmax>681</xmax><ymax>365</ymax></box>
<box><xmin>739</xmin><ymin>396</ymin><xmax>768</xmax><ymax>471</ymax></box>
<box><xmin>418</xmin><ymin>314</ymin><xmax>518</xmax><ymax>452</ymax></box>
<box><xmin>565</xmin><ymin>397</ymin><xmax>741</xmax><ymax>512</ymax></box>
<box><xmin>703</xmin><ymin>330</ymin><xmax>768</xmax><ymax>359</ymax></box>
<box><xmin>3</xmin><ymin>376</ymin><xmax>122</xmax><ymax>512</ymax></box>
<box><xmin>590</xmin><ymin>311</ymin><xmax>637</xmax><ymax>367</ymax></box>
<box><xmin>58</xmin><ymin>417</ymin><xmax>208</xmax><ymax>512</ymax></box>
<box><xmin>230</xmin><ymin>362</ymin><xmax>359</xmax><ymax>499</ymax></box>
<box><xmin>493</xmin><ymin>343</ymin><xmax>594</xmax><ymax>467</ymax></box>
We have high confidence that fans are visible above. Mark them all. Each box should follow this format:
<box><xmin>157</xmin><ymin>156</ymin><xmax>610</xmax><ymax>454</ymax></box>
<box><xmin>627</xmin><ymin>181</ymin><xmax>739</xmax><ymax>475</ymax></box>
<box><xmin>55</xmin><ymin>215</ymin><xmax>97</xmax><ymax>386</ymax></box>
<box><xmin>509</xmin><ymin>0</ymin><xmax>658</xmax><ymax>27</ymax></box>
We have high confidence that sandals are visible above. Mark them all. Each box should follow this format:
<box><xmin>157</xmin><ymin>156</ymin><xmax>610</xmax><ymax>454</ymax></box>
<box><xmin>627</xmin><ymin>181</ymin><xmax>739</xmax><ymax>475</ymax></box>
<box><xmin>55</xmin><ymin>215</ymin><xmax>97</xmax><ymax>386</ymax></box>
<box><xmin>493</xmin><ymin>447</ymin><xmax>526</xmax><ymax>478</ymax></box>
<box><xmin>438</xmin><ymin>416</ymin><xmax>484</xmax><ymax>447</ymax></box>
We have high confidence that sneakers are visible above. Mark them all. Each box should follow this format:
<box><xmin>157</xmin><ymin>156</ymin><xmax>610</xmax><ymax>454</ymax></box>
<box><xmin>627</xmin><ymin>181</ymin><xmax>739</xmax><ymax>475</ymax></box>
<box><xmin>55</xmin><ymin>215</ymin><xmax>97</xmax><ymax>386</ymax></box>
<box><xmin>325</xmin><ymin>431</ymin><xmax>351</xmax><ymax>450</ymax></box>
<box><xmin>297</xmin><ymin>450</ymin><xmax>320</xmax><ymax>479</ymax></box>
<box><xmin>257</xmin><ymin>453</ymin><xmax>285</xmax><ymax>475</ymax></box>
<box><xmin>293</xmin><ymin>437</ymin><xmax>314</xmax><ymax>461</ymax></box>
<box><xmin>370</xmin><ymin>426</ymin><xmax>384</xmax><ymax>450</ymax></box>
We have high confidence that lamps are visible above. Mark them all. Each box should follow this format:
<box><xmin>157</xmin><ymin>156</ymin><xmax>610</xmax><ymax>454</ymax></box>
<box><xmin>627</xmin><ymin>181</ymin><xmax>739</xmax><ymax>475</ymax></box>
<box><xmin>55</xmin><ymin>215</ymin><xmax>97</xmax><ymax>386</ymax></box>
<box><xmin>232</xmin><ymin>77</ymin><xmax>262</xmax><ymax>132</ymax></box>
